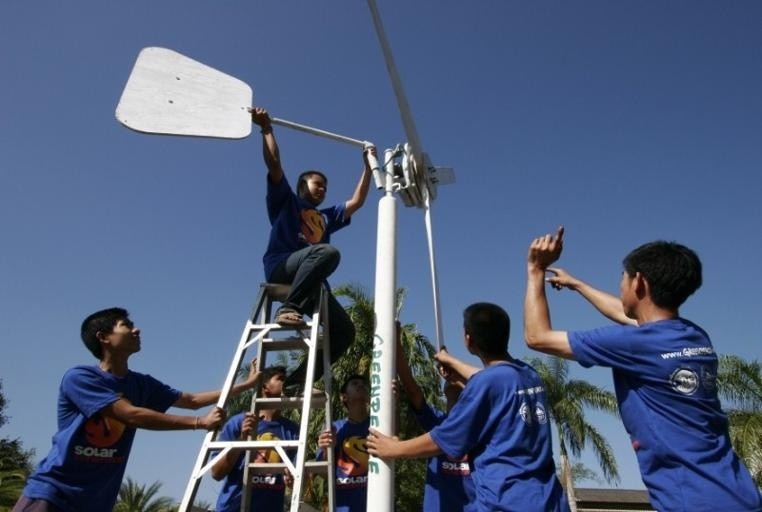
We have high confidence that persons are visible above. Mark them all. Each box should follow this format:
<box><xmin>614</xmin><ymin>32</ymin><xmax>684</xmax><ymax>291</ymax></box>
<box><xmin>311</xmin><ymin>376</ymin><xmax>401</xmax><ymax>512</ymax></box>
<box><xmin>11</xmin><ymin>305</ymin><xmax>262</xmax><ymax>511</ymax></box>
<box><xmin>363</xmin><ymin>300</ymin><xmax>570</xmax><ymax>512</ymax></box>
<box><xmin>250</xmin><ymin>104</ymin><xmax>380</xmax><ymax>396</ymax></box>
<box><xmin>209</xmin><ymin>363</ymin><xmax>301</xmax><ymax>512</ymax></box>
<box><xmin>521</xmin><ymin>222</ymin><xmax>762</xmax><ymax>511</ymax></box>
<box><xmin>393</xmin><ymin>320</ymin><xmax>478</xmax><ymax>512</ymax></box>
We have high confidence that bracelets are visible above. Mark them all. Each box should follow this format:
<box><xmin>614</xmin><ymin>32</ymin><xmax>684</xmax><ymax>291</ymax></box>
<box><xmin>194</xmin><ymin>416</ymin><xmax>200</xmax><ymax>429</ymax></box>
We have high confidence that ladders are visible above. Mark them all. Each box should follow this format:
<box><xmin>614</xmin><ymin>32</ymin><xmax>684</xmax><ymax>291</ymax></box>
<box><xmin>178</xmin><ymin>279</ymin><xmax>337</xmax><ymax>512</ymax></box>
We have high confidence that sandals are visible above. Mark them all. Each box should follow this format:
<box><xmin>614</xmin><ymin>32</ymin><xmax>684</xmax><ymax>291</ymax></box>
<box><xmin>274</xmin><ymin>307</ymin><xmax>308</xmax><ymax>329</ymax></box>
<box><xmin>280</xmin><ymin>386</ymin><xmax>327</xmax><ymax>399</ymax></box>
